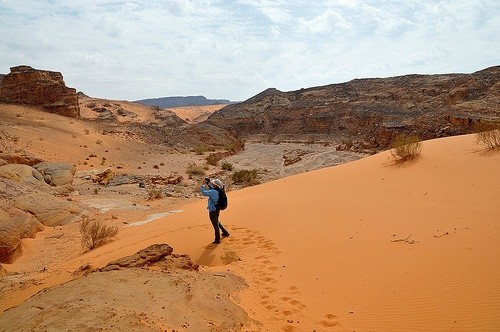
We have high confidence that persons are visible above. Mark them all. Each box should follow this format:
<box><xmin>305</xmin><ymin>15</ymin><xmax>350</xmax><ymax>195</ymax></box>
<box><xmin>201</xmin><ymin>177</ymin><xmax>230</xmax><ymax>244</ymax></box>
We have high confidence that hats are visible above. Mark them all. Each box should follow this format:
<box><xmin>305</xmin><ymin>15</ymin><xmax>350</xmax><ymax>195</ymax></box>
<box><xmin>212</xmin><ymin>179</ymin><xmax>222</xmax><ymax>188</ymax></box>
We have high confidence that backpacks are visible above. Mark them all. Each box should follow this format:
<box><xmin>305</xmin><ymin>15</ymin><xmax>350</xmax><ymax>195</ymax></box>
<box><xmin>210</xmin><ymin>187</ymin><xmax>227</xmax><ymax>210</ymax></box>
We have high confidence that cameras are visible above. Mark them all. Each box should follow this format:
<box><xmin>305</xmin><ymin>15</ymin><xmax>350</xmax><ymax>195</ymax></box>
<box><xmin>205</xmin><ymin>178</ymin><xmax>208</xmax><ymax>183</ymax></box>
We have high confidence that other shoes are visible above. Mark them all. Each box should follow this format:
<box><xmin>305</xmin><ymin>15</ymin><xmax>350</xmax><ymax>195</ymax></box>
<box><xmin>213</xmin><ymin>241</ymin><xmax>220</xmax><ymax>244</ymax></box>
<box><xmin>221</xmin><ymin>233</ymin><xmax>230</xmax><ymax>236</ymax></box>
